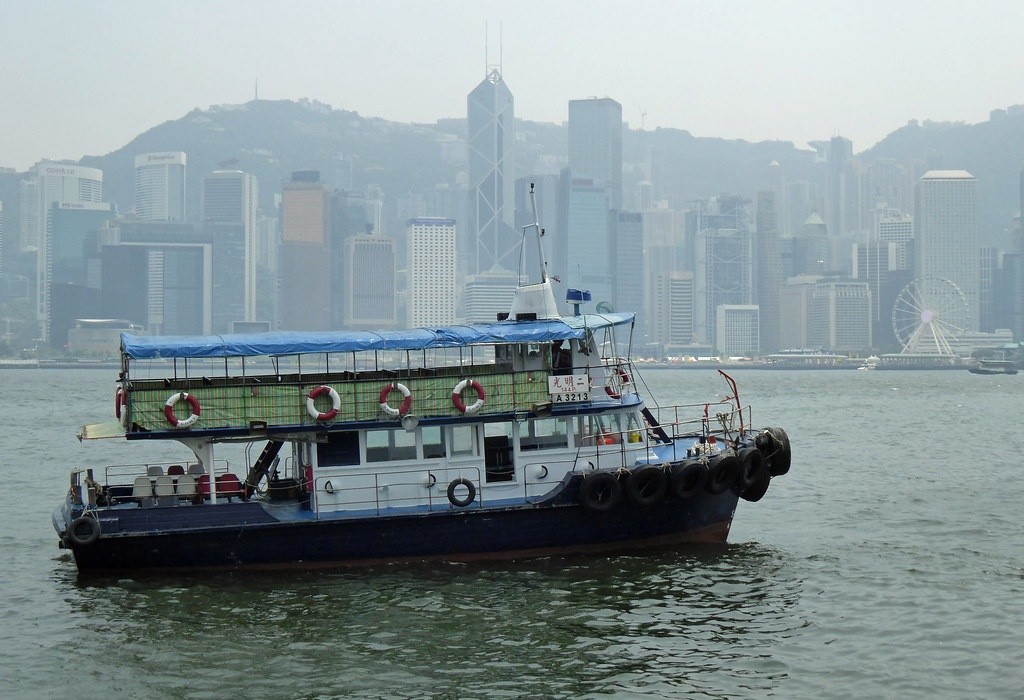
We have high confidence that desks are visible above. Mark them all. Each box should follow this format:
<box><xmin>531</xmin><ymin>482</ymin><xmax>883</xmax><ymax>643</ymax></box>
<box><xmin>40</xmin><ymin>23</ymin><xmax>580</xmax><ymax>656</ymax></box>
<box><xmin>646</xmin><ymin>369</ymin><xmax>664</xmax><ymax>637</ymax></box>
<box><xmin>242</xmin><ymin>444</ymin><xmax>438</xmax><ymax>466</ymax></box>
<box><xmin>137</xmin><ymin>472</ymin><xmax>238</xmax><ymax>483</ymax></box>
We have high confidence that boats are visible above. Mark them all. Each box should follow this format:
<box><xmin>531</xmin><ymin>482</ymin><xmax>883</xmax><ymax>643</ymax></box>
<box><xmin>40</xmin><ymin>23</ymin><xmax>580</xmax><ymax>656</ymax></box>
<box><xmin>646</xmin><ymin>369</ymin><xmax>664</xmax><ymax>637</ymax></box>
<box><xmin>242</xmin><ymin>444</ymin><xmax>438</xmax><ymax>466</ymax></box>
<box><xmin>51</xmin><ymin>183</ymin><xmax>792</xmax><ymax>583</ymax></box>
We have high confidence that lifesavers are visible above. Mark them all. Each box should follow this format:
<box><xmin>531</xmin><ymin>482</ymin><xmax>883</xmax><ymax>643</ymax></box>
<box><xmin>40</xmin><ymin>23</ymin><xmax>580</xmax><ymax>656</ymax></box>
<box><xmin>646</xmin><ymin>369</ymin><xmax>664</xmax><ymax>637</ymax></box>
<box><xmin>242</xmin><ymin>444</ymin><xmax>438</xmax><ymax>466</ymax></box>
<box><xmin>447</xmin><ymin>478</ymin><xmax>476</xmax><ymax>507</ymax></box>
<box><xmin>305</xmin><ymin>467</ymin><xmax>313</xmax><ymax>492</ymax></box>
<box><xmin>306</xmin><ymin>385</ymin><xmax>341</xmax><ymax>421</ymax></box>
<box><xmin>576</xmin><ymin>427</ymin><xmax>791</xmax><ymax>519</ymax></box>
<box><xmin>604</xmin><ymin>369</ymin><xmax>629</xmax><ymax>399</ymax></box>
<box><xmin>68</xmin><ymin>517</ymin><xmax>100</xmax><ymax>547</ymax></box>
<box><xmin>115</xmin><ymin>385</ymin><xmax>127</xmax><ymax>428</ymax></box>
<box><xmin>379</xmin><ymin>382</ymin><xmax>412</xmax><ymax>416</ymax></box>
<box><xmin>452</xmin><ymin>379</ymin><xmax>485</xmax><ymax>413</ymax></box>
<box><xmin>164</xmin><ymin>392</ymin><xmax>201</xmax><ymax>428</ymax></box>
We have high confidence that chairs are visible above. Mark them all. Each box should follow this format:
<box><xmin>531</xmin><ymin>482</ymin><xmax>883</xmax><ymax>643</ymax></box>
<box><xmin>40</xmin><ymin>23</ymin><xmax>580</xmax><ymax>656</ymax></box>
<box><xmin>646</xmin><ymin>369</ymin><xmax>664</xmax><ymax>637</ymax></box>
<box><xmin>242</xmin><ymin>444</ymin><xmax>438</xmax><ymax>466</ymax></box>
<box><xmin>188</xmin><ymin>465</ymin><xmax>205</xmax><ymax>475</ymax></box>
<box><xmin>132</xmin><ymin>478</ymin><xmax>153</xmax><ymax>508</ymax></box>
<box><xmin>168</xmin><ymin>466</ymin><xmax>185</xmax><ymax>475</ymax></box>
<box><xmin>147</xmin><ymin>466</ymin><xmax>164</xmax><ymax>476</ymax></box>
<box><xmin>175</xmin><ymin>476</ymin><xmax>196</xmax><ymax>505</ymax></box>
<box><xmin>196</xmin><ymin>475</ymin><xmax>217</xmax><ymax>500</ymax></box>
<box><xmin>219</xmin><ymin>474</ymin><xmax>243</xmax><ymax>501</ymax></box>
<box><xmin>155</xmin><ymin>476</ymin><xmax>174</xmax><ymax>495</ymax></box>
